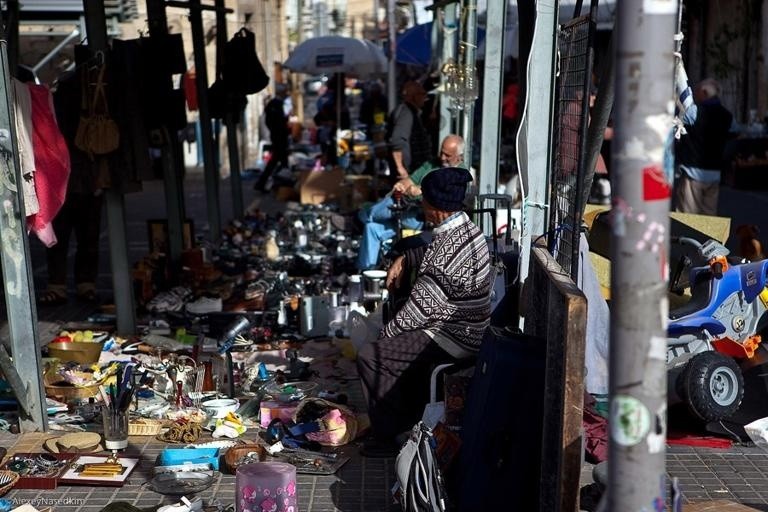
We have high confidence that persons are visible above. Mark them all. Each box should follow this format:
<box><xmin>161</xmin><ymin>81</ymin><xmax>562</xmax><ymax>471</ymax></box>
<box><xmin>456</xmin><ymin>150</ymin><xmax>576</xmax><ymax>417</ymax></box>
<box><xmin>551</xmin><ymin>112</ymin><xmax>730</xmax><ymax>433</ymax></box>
<box><xmin>559</xmin><ymin>81</ymin><xmax>615</xmax><ymax>178</ymax></box>
<box><xmin>331</xmin><ymin>136</ymin><xmax>469</xmax><ymax>275</ymax></box>
<box><xmin>253</xmin><ymin>85</ymin><xmax>289</xmax><ymax>194</ymax></box>
<box><xmin>313</xmin><ymin>80</ymin><xmax>388</xmax><ymax>155</ymax></box>
<box><xmin>676</xmin><ymin>78</ymin><xmax>733</xmax><ymax>217</ymax></box>
<box><xmin>383</xmin><ymin>81</ymin><xmax>433</xmax><ymax>187</ymax></box>
<box><xmin>357</xmin><ymin>167</ymin><xmax>493</xmax><ymax>458</ymax></box>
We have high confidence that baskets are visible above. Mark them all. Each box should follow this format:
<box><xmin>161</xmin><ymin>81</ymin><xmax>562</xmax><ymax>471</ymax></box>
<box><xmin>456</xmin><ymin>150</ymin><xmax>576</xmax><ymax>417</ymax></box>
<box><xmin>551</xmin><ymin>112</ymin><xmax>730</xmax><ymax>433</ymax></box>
<box><xmin>225</xmin><ymin>444</ymin><xmax>266</xmax><ymax>475</ymax></box>
<box><xmin>44</xmin><ymin>372</ymin><xmax>99</xmax><ymax>398</ymax></box>
<box><xmin>128</xmin><ymin>419</ymin><xmax>163</xmax><ymax>436</ymax></box>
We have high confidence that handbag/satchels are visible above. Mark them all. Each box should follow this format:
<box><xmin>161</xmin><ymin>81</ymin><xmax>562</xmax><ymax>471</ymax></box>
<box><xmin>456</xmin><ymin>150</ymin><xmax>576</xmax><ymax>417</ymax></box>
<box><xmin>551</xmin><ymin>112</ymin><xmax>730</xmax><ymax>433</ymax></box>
<box><xmin>395</xmin><ymin>421</ymin><xmax>448</xmax><ymax>512</ymax></box>
<box><xmin>73</xmin><ymin>116</ymin><xmax>120</xmax><ymax>154</ymax></box>
<box><xmin>291</xmin><ymin>397</ymin><xmax>359</xmax><ymax>447</ymax></box>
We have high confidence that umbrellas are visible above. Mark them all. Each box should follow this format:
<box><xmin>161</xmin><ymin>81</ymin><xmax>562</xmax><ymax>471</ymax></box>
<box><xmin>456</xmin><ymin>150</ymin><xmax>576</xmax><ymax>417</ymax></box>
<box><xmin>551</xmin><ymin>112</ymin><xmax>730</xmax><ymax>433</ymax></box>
<box><xmin>282</xmin><ymin>35</ymin><xmax>389</xmax><ymax>130</ymax></box>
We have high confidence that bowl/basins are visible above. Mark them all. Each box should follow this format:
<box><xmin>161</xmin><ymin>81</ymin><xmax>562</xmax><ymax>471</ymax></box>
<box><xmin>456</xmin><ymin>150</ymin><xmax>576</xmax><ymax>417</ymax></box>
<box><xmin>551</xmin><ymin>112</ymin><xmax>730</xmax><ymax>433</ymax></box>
<box><xmin>47</xmin><ymin>342</ymin><xmax>103</xmax><ymax>364</ymax></box>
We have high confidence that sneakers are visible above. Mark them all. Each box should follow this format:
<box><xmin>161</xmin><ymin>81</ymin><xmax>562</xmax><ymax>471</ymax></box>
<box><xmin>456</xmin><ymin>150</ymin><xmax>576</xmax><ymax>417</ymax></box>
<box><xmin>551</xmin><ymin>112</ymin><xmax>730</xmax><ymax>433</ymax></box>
<box><xmin>38</xmin><ymin>292</ymin><xmax>68</xmax><ymax>304</ymax></box>
<box><xmin>84</xmin><ymin>288</ymin><xmax>99</xmax><ymax>299</ymax></box>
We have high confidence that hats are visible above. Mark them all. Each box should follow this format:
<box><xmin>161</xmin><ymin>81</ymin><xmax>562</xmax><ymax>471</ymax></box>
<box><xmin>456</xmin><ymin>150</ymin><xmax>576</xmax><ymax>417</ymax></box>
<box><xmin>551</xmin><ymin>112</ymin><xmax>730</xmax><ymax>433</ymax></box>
<box><xmin>421</xmin><ymin>168</ymin><xmax>473</xmax><ymax>212</ymax></box>
